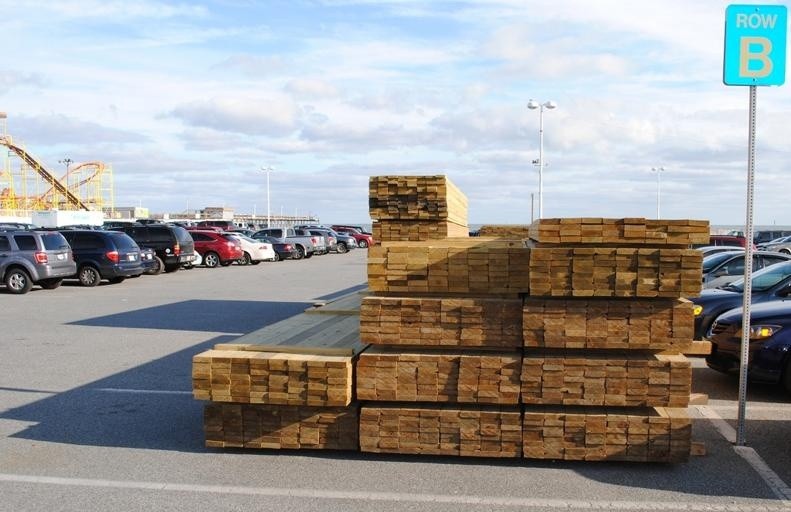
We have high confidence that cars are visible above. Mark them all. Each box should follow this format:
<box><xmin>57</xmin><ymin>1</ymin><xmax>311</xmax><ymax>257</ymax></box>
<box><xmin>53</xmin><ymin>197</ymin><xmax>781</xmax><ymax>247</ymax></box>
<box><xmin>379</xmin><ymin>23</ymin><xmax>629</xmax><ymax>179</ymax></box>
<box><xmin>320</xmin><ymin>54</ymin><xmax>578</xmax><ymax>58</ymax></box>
<box><xmin>691</xmin><ymin>227</ymin><xmax>791</xmax><ymax>356</ymax></box>
<box><xmin>701</xmin><ymin>298</ymin><xmax>791</xmax><ymax>396</ymax></box>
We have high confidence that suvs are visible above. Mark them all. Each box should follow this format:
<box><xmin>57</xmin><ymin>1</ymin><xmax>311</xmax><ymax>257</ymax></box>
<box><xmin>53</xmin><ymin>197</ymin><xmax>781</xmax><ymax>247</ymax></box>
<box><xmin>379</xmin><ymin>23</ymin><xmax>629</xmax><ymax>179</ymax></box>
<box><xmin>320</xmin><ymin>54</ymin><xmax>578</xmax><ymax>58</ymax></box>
<box><xmin>1</xmin><ymin>229</ymin><xmax>78</xmax><ymax>295</ymax></box>
<box><xmin>55</xmin><ymin>229</ymin><xmax>143</xmax><ymax>287</ymax></box>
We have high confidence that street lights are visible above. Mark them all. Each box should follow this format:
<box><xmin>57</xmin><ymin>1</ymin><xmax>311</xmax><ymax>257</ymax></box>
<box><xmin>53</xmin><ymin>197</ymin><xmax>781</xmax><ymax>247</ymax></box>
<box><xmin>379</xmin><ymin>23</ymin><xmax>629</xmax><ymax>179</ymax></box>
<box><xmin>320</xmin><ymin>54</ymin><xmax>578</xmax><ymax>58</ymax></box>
<box><xmin>261</xmin><ymin>166</ymin><xmax>271</xmax><ymax>229</ymax></box>
<box><xmin>60</xmin><ymin>157</ymin><xmax>74</xmax><ymax>210</ymax></box>
<box><xmin>526</xmin><ymin>98</ymin><xmax>559</xmax><ymax>219</ymax></box>
<box><xmin>651</xmin><ymin>165</ymin><xmax>664</xmax><ymax>219</ymax></box>
<box><xmin>530</xmin><ymin>157</ymin><xmax>551</xmax><ymax>218</ymax></box>
